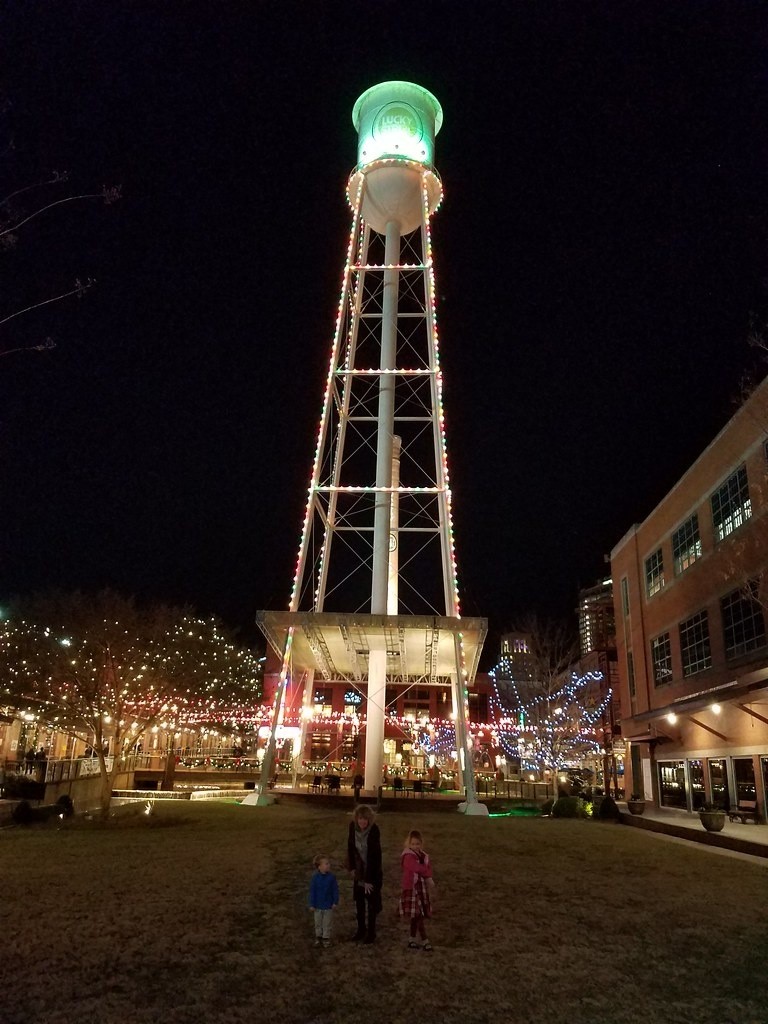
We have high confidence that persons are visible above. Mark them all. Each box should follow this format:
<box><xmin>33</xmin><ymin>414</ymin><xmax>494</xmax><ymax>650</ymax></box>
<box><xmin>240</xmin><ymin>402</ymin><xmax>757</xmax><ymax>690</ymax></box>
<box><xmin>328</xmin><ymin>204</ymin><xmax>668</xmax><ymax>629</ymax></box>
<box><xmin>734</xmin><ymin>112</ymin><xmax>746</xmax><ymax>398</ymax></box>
<box><xmin>35</xmin><ymin>747</ymin><xmax>45</xmax><ymax>760</ymax></box>
<box><xmin>396</xmin><ymin>831</ymin><xmax>435</xmax><ymax>952</ymax></box>
<box><xmin>85</xmin><ymin>743</ymin><xmax>142</xmax><ymax>758</ymax></box>
<box><xmin>345</xmin><ymin>804</ymin><xmax>384</xmax><ymax>945</ymax></box>
<box><xmin>307</xmin><ymin>854</ymin><xmax>339</xmax><ymax>948</ymax></box>
<box><xmin>24</xmin><ymin>747</ymin><xmax>35</xmax><ymax>774</ymax></box>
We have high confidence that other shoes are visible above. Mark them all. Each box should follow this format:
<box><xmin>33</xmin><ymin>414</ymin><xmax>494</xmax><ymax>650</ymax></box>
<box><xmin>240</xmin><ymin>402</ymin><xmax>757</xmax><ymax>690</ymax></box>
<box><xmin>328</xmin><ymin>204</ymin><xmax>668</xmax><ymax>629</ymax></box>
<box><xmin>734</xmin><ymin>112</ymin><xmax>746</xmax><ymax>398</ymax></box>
<box><xmin>313</xmin><ymin>939</ymin><xmax>320</xmax><ymax>947</ymax></box>
<box><xmin>369</xmin><ymin>935</ymin><xmax>375</xmax><ymax>942</ymax></box>
<box><xmin>321</xmin><ymin>940</ymin><xmax>332</xmax><ymax>948</ymax></box>
<box><xmin>352</xmin><ymin>934</ymin><xmax>363</xmax><ymax>941</ymax></box>
<box><xmin>424</xmin><ymin>943</ymin><xmax>433</xmax><ymax>951</ymax></box>
<box><xmin>408</xmin><ymin>941</ymin><xmax>417</xmax><ymax>948</ymax></box>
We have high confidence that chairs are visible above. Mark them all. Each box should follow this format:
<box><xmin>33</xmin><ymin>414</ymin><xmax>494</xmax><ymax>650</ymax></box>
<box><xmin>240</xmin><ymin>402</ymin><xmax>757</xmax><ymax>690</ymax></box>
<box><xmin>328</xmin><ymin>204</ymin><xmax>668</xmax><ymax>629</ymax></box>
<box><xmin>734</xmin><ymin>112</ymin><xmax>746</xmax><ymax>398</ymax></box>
<box><xmin>307</xmin><ymin>773</ymin><xmax>440</xmax><ymax>798</ymax></box>
<box><xmin>267</xmin><ymin>773</ymin><xmax>278</xmax><ymax>789</ymax></box>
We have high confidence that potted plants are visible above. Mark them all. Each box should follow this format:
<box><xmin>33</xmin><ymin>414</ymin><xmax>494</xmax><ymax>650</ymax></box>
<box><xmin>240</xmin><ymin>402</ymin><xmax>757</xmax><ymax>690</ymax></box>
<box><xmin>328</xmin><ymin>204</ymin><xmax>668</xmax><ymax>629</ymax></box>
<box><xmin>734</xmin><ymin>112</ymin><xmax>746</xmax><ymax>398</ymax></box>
<box><xmin>628</xmin><ymin>793</ymin><xmax>645</xmax><ymax>814</ymax></box>
<box><xmin>699</xmin><ymin>800</ymin><xmax>727</xmax><ymax>831</ymax></box>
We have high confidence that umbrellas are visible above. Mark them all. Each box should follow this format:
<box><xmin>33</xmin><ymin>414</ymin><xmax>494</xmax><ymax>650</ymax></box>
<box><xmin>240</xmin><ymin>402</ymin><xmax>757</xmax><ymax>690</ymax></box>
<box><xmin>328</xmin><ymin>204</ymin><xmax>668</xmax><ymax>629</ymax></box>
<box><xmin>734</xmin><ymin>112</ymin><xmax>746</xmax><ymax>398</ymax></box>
<box><xmin>558</xmin><ymin>767</ymin><xmax>625</xmax><ymax>775</ymax></box>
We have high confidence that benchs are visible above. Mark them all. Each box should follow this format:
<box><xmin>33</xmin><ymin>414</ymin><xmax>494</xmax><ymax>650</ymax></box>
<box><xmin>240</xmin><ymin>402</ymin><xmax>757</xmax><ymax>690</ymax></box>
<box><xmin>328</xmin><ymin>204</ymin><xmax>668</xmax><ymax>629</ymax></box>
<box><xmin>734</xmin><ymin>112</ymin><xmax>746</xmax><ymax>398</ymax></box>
<box><xmin>730</xmin><ymin>800</ymin><xmax>758</xmax><ymax>823</ymax></box>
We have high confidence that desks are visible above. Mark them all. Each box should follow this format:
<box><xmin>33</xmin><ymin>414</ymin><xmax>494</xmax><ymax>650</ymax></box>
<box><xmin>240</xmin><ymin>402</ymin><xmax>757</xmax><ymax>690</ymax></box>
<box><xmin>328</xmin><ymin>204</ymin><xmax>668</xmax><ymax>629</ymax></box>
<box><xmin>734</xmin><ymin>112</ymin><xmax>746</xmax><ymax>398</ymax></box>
<box><xmin>504</xmin><ymin>779</ymin><xmax>519</xmax><ymax>797</ymax></box>
<box><xmin>521</xmin><ymin>781</ymin><xmax>550</xmax><ymax>799</ymax></box>
